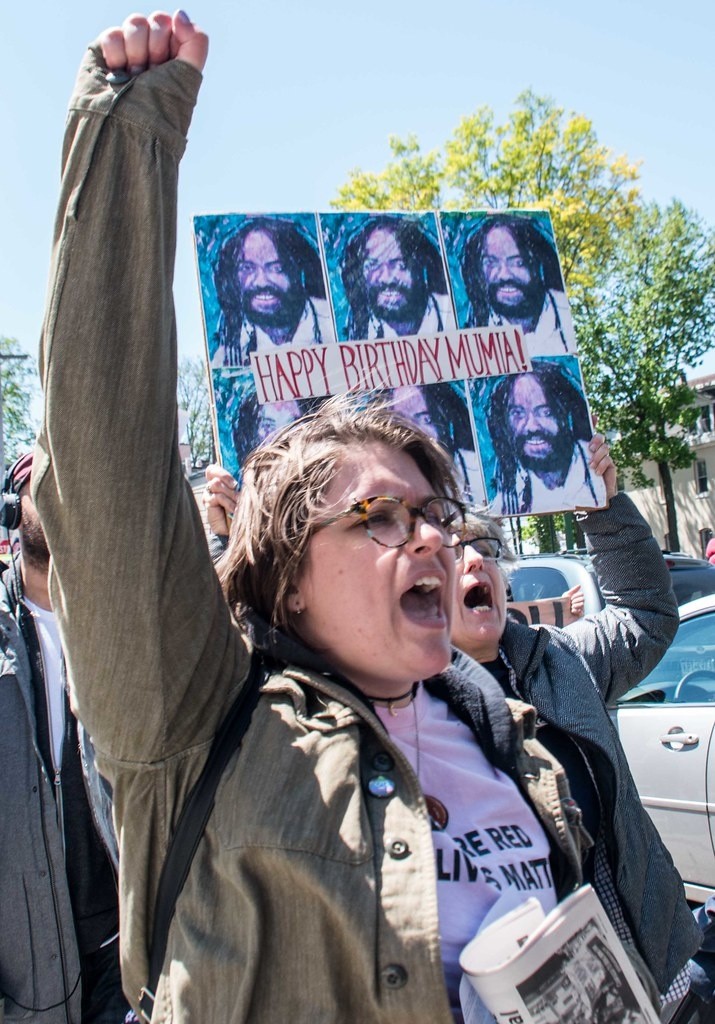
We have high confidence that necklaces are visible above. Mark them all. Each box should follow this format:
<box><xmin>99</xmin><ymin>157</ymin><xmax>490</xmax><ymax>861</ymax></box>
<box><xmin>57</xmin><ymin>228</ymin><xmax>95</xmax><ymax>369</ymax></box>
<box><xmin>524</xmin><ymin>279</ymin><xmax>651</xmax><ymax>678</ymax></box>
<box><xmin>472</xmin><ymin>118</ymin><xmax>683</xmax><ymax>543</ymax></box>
<box><xmin>368</xmin><ymin>697</ymin><xmax>448</xmax><ymax>833</ymax></box>
<box><xmin>367</xmin><ymin>689</ymin><xmax>412</xmax><ymax>717</ymax></box>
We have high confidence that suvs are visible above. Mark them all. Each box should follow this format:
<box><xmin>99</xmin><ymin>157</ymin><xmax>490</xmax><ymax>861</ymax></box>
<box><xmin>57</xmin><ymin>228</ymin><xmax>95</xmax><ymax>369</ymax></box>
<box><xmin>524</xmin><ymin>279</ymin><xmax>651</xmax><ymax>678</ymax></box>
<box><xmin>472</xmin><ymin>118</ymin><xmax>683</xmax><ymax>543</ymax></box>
<box><xmin>506</xmin><ymin>548</ymin><xmax>715</xmax><ymax>638</ymax></box>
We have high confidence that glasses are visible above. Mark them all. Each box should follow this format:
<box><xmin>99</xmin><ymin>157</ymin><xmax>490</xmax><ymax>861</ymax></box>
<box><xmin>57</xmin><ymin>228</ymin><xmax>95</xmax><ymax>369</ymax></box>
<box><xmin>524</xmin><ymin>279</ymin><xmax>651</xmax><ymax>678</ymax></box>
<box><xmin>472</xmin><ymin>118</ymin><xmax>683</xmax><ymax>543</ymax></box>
<box><xmin>452</xmin><ymin>537</ymin><xmax>507</xmax><ymax>564</ymax></box>
<box><xmin>307</xmin><ymin>495</ymin><xmax>466</xmax><ymax>549</ymax></box>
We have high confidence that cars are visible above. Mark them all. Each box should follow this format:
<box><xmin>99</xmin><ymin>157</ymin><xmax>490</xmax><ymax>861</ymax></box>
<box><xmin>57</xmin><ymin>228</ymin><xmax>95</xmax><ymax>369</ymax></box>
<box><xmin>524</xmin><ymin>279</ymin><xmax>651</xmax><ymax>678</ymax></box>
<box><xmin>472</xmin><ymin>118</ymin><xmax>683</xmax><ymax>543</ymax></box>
<box><xmin>605</xmin><ymin>595</ymin><xmax>715</xmax><ymax>916</ymax></box>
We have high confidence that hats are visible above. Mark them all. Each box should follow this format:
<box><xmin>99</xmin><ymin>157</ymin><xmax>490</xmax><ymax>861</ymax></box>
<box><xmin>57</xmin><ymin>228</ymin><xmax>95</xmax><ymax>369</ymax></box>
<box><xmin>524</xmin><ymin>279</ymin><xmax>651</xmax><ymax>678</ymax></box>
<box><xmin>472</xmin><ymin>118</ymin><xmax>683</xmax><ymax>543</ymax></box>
<box><xmin>11</xmin><ymin>454</ymin><xmax>34</xmax><ymax>494</ymax></box>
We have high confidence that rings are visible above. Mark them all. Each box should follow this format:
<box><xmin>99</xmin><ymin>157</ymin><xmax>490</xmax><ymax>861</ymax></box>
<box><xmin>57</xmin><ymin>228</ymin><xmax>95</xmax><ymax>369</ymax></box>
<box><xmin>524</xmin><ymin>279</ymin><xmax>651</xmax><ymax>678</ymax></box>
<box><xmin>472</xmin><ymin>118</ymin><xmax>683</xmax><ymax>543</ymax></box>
<box><xmin>205</xmin><ymin>485</ymin><xmax>211</xmax><ymax>493</ymax></box>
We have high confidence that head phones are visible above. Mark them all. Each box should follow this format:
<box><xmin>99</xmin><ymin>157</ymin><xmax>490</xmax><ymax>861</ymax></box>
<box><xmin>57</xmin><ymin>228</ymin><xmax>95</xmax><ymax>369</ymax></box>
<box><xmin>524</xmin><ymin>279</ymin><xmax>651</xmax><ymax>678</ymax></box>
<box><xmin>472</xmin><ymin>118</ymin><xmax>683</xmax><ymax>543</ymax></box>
<box><xmin>0</xmin><ymin>451</ymin><xmax>32</xmax><ymax>530</ymax></box>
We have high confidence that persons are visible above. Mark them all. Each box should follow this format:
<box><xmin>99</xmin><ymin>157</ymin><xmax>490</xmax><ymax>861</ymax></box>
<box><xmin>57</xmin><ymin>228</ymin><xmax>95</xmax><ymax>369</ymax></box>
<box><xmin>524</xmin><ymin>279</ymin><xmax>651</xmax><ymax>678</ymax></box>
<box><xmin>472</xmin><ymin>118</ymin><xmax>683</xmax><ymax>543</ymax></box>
<box><xmin>30</xmin><ymin>8</ymin><xmax>659</xmax><ymax>1024</ymax></box>
<box><xmin>0</xmin><ymin>452</ymin><xmax>137</xmax><ymax>1024</ymax></box>
<box><xmin>560</xmin><ymin>584</ymin><xmax>585</xmax><ymax>617</ymax></box>
<box><xmin>705</xmin><ymin>538</ymin><xmax>715</xmax><ymax>565</ymax></box>
<box><xmin>201</xmin><ymin>413</ymin><xmax>715</xmax><ymax>1024</ymax></box>
